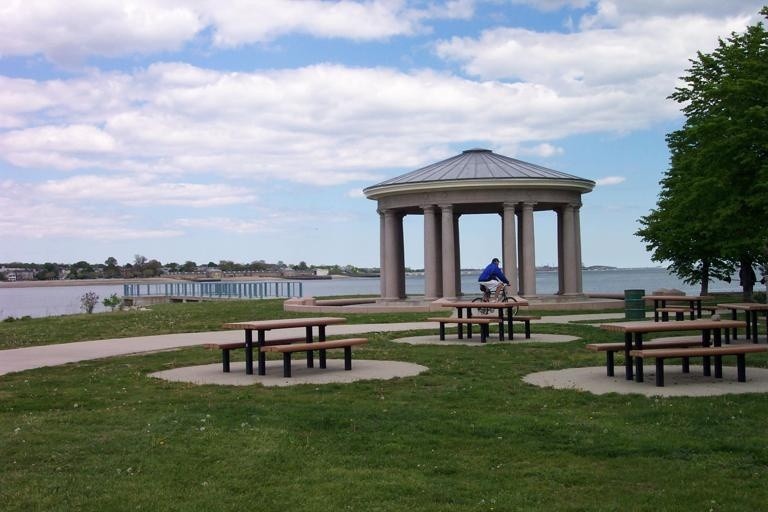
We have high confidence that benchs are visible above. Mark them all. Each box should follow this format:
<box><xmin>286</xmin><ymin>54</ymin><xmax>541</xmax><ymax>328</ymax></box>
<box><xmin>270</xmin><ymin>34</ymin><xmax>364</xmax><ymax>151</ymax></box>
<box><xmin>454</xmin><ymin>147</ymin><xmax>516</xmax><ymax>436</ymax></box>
<box><xmin>655</xmin><ymin>304</ymin><xmax>718</xmax><ymax>320</ymax></box>
<box><xmin>427</xmin><ymin>314</ymin><xmax>542</xmax><ymax>343</ymax></box>
<box><xmin>585</xmin><ymin>340</ymin><xmax>768</xmax><ymax>387</ymax></box>
<box><xmin>202</xmin><ymin>336</ymin><xmax>370</xmax><ymax>378</ymax></box>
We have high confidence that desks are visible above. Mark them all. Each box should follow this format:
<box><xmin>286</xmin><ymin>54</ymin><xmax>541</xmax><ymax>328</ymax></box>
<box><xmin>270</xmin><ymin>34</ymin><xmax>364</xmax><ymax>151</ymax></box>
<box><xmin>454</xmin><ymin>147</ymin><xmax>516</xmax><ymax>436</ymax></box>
<box><xmin>640</xmin><ymin>293</ymin><xmax>716</xmax><ymax>321</ymax></box>
<box><xmin>222</xmin><ymin>317</ymin><xmax>347</xmax><ymax>375</ymax></box>
<box><xmin>600</xmin><ymin>318</ymin><xmax>747</xmax><ymax>383</ymax></box>
<box><xmin>717</xmin><ymin>302</ymin><xmax>768</xmax><ymax>343</ymax></box>
<box><xmin>441</xmin><ymin>302</ymin><xmax>529</xmax><ymax>340</ymax></box>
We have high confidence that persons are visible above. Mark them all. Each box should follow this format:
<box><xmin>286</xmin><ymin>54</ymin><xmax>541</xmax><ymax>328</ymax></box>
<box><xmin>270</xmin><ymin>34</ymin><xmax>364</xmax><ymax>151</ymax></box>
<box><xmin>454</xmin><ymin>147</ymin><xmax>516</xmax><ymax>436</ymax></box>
<box><xmin>477</xmin><ymin>258</ymin><xmax>511</xmax><ymax>314</ymax></box>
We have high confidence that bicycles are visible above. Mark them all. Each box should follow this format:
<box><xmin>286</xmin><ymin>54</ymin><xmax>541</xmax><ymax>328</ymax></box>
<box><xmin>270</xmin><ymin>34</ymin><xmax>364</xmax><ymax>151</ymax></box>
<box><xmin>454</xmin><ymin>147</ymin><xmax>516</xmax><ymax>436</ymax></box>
<box><xmin>472</xmin><ymin>284</ymin><xmax>519</xmax><ymax>316</ymax></box>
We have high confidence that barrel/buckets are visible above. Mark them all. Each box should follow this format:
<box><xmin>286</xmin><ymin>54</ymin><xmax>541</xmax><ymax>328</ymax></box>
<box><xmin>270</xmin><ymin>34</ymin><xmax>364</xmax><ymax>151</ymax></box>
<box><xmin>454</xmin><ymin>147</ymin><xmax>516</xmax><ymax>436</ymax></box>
<box><xmin>624</xmin><ymin>288</ymin><xmax>646</xmax><ymax>321</ymax></box>
